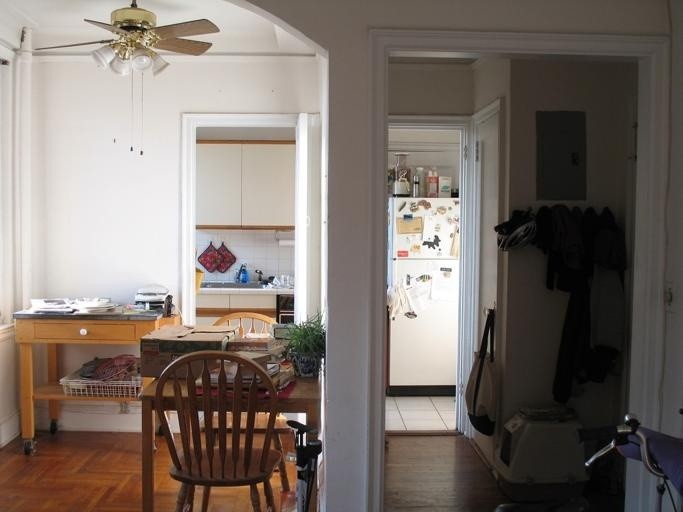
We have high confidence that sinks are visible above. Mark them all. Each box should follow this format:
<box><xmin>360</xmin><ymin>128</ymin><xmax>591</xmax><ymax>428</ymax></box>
<box><xmin>200</xmin><ymin>282</ymin><xmax>259</xmax><ymax>289</ymax></box>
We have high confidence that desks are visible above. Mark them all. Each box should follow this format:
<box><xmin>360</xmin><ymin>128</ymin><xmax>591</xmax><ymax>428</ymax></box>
<box><xmin>10</xmin><ymin>305</ymin><xmax>163</xmax><ymax>440</ymax></box>
<box><xmin>137</xmin><ymin>363</ymin><xmax>320</xmax><ymax>511</ymax></box>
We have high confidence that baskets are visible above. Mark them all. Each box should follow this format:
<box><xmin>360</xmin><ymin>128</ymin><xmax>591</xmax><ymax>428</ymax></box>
<box><xmin>60</xmin><ymin>352</ymin><xmax>143</xmax><ymax>398</ymax></box>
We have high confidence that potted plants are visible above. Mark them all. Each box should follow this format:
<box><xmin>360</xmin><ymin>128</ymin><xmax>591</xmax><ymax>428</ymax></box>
<box><xmin>282</xmin><ymin>310</ymin><xmax>324</xmax><ymax>378</ymax></box>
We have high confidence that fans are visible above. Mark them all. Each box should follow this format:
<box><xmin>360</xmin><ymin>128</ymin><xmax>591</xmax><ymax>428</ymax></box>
<box><xmin>32</xmin><ymin>0</ymin><xmax>221</xmax><ymax>58</ymax></box>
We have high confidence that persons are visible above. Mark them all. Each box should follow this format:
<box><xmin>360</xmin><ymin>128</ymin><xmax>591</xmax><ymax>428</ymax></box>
<box><xmin>193</xmin><ymin>336</ymin><xmax>295</xmax><ymax>392</ymax></box>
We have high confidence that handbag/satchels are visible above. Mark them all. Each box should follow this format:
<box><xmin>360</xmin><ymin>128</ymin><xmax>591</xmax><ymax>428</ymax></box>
<box><xmin>465</xmin><ymin>350</ymin><xmax>498</xmax><ymax>435</ymax></box>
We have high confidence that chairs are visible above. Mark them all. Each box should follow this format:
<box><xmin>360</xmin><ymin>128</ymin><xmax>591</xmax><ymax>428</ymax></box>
<box><xmin>154</xmin><ymin>312</ymin><xmax>292</xmax><ymax>512</ymax></box>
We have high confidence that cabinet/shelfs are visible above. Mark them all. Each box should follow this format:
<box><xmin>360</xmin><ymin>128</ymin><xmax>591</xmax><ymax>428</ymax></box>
<box><xmin>195</xmin><ymin>294</ymin><xmax>276</xmax><ymax>333</ymax></box>
<box><xmin>195</xmin><ymin>143</ymin><xmax>295</xmax><ymax>226</ymax></box>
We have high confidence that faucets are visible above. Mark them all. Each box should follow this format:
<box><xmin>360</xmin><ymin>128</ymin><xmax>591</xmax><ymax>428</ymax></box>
<box><xmin>235</xmin><ymin>264</ymin><xmax>246</xmax><ymax>282</ymax></box>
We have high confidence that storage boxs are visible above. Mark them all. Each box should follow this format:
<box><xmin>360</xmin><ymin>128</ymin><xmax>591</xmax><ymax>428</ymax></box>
<box><xmin>139</xmin><ymin>323</ymin><xmax>238</xmax><ymax>380</ymax></box>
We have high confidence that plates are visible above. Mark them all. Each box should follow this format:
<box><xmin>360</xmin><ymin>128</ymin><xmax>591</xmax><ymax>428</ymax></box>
<box><xmin>70</xmin><ymin>304</ymin><xmax>115</xmax><ymax>314</ymax></box>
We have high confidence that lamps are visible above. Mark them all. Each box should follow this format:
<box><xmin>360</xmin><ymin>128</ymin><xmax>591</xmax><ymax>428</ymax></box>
<box><xmin>89</xmin><ymin>31</ymin><xmax>171</xmax><ymax>79</ymax></box>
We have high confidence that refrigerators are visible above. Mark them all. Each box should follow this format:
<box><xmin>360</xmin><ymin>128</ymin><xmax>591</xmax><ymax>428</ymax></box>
<box><xmin>386</xmin><ymin>197</ymin><xmax>459</xmax><ymax>397</ymax></box>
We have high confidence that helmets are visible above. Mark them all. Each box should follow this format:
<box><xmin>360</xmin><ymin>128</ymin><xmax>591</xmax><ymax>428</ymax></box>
<box><xmin>495</xmin><ymin>212</ymin><xmax>536</xmax><ymax>251</ymax></box>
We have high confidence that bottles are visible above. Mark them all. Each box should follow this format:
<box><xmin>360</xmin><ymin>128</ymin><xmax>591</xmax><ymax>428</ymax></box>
<box><xmin>392</xmin><ymin>153</ymin><xmax>437</xmax><ymax>197</ymax></box>
<box><xmin>240</xmin><ymin>266</ymin><xmax>249</xmax><ymax>284</ymax></box>
